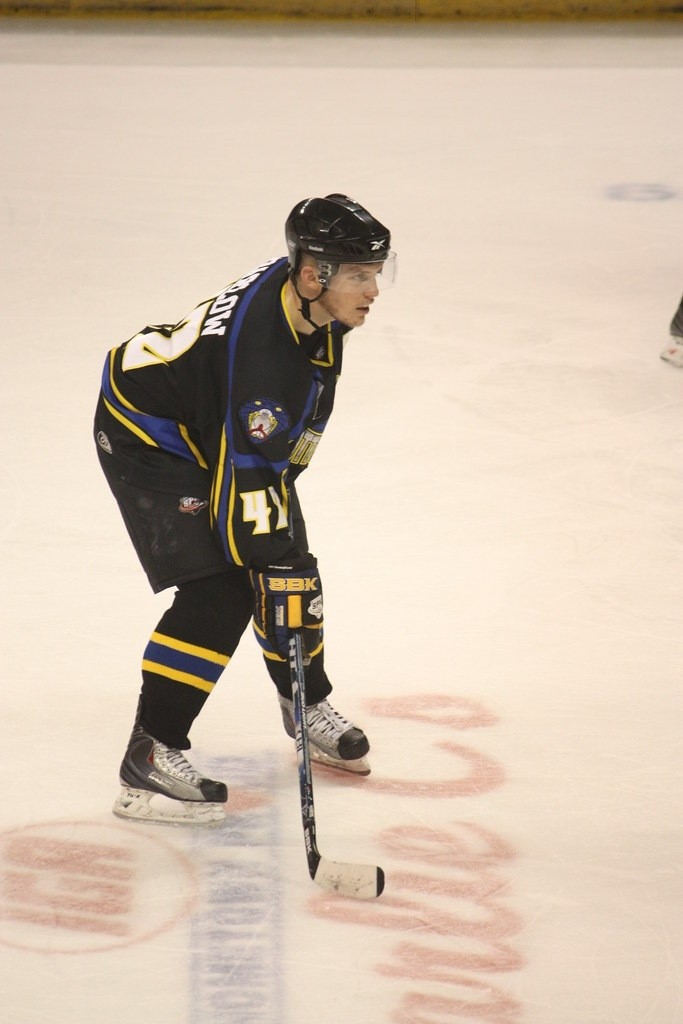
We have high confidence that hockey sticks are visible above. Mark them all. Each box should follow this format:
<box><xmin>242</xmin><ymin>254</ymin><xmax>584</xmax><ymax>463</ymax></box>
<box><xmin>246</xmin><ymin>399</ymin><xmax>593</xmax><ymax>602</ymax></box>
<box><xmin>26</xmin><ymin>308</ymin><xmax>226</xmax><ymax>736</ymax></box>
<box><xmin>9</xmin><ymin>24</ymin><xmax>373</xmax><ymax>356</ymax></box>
<box><xmin>286</xmin><ymin>481</ymin><xmax>390</xmax><ymax>901</ymax></box>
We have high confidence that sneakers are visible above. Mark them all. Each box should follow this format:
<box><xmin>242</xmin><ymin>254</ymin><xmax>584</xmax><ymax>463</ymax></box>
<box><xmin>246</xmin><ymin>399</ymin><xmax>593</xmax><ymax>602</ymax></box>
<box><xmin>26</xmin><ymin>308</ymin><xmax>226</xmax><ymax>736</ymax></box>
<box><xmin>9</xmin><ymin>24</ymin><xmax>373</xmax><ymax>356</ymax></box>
<box><xmin>113</xmin><ymin>694</ymin><xmax>227</xmax><ymax>830</ymax></box>
<box><xmin>278</xmin><ymin>691</ymin><xmax>372</xmax><ymax>777</ymax></box>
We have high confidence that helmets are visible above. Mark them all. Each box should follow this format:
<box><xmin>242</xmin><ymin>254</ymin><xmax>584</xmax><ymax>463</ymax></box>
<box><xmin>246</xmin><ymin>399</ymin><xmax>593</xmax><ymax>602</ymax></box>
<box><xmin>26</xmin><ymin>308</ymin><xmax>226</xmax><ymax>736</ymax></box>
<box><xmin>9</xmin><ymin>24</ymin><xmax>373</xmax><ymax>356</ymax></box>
<box><xmin>283</xmin><ymin>194</ymin><xmax>390</xmax><ymax>271</ymax></box>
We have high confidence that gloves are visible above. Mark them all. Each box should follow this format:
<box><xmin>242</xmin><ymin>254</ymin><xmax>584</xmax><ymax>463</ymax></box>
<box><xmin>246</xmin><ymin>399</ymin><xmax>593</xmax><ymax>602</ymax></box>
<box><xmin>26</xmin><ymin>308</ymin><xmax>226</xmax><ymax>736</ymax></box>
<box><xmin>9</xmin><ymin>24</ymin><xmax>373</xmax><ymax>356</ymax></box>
<box><xmin>248</xmin><ymin>553</ymin><xmax>323</xmax><ymax>660</ymax></box>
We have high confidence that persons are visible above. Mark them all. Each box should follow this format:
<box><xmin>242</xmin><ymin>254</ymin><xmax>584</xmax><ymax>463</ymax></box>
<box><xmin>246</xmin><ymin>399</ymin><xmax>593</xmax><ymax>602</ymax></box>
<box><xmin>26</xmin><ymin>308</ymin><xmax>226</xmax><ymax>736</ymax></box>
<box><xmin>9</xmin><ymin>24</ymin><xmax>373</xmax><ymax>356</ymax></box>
<box><xmin>93</xmin><ymin>193</ymin><xmax>398</xmax><ymax>826</ymax></box>
